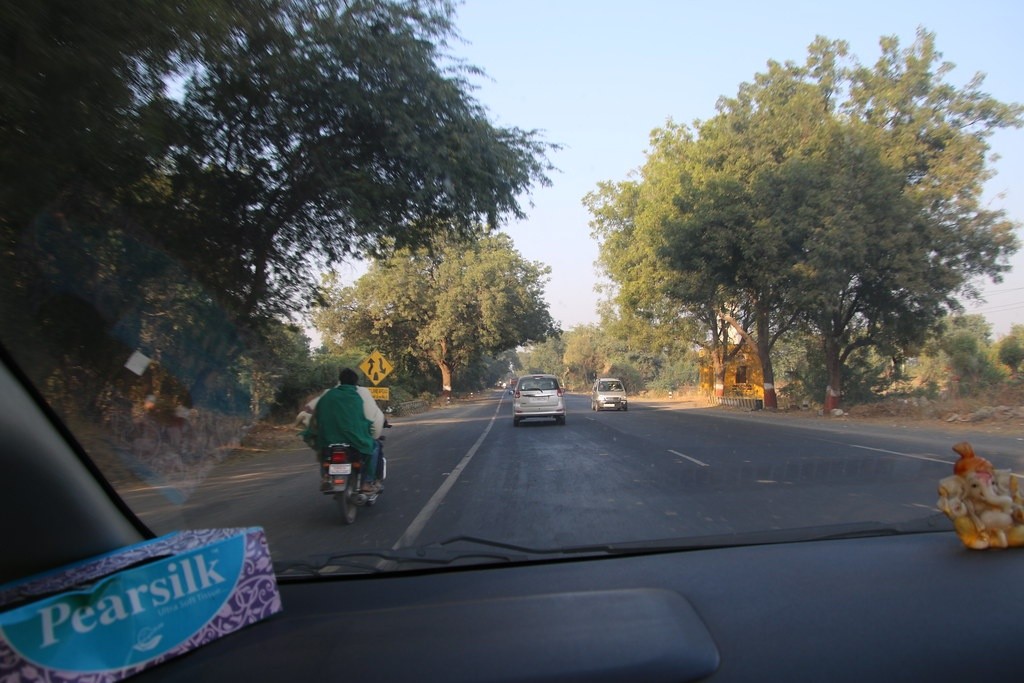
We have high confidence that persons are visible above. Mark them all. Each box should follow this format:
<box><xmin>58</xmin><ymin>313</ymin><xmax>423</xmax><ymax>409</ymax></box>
<box><xmin>612</xmin><ymin>384</ymin><xmax>619</xmax><ymax>392</ymax></box>
<box><xmin>599</xmin><ymin>384</ymin><xmax>605</xmax><ymax>391</ymax></box>
<box><xmin>297</xmin><ymin>366</ymin><xmax>385</xmax><ymax>495</ymax></box>
<box><xmin>499</xmin><ymin>379</ymin><xmax>501</xmax><ymax>386</ymax></box>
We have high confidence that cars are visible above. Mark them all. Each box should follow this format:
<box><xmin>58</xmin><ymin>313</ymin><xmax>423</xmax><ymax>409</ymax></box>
<box><xmin>509</xmin><ymin>374</ymin><xmax>566</xmax><ymax>426</ymax></box>
<box><xmin>590</xmin><ymin>378</ymin><xmax>627</xmax><ymax>411</ymax></box>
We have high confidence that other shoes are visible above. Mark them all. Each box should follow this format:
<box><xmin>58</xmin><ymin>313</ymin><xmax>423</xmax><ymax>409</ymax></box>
<box><xmin>320</xmin><ymin>474</ymin><xmax>330</xmax><ymax>491</ymax></box>
<box><xmin>375</xmin><ymin>479</ymin><xmax>383</xmax><ymax>487</ymax></box>
<box><xmin>360</xmin><ymin>482</ymin><xmax>372</xmax><ymax>491</ymax></box>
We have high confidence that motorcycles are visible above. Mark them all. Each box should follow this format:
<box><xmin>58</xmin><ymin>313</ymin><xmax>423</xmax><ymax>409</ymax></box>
<box><xmin>319</xmin><ymin>423</ymin><xmax>391</xmax><ymax>526</ymax></box>
